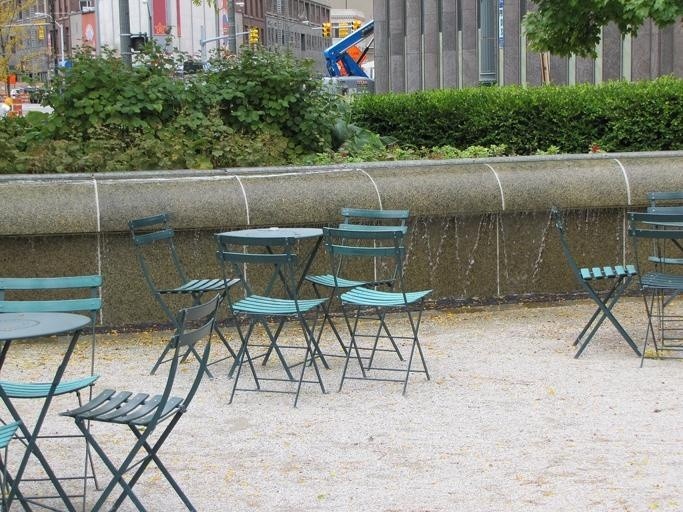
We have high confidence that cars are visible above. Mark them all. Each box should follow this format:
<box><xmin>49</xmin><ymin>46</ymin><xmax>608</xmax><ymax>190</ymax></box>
<box><xmin>9</xmin><ymin>88</ymin><xmax>55</xmax><ymax>104</ymax></box>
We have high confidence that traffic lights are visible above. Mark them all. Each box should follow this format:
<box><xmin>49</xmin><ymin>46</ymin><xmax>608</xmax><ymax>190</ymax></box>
<box><xmin>321</xmin><ymin>23</ymin><xmax>331</xmax><ymax>37</ymax></box>
<box><xmin>249</xmin><ymin>28</ymin><xmax>259</xmax><ymax>44</ymax></box>
<box><xmin>132</xmin><ymin>36</ymin><xmax>147</xmax><ymax>51</ymax></box>
<box><xmin>351</xmin><ymin>21</ymin><xmax>361</xmax><ymax>34</ymax></box>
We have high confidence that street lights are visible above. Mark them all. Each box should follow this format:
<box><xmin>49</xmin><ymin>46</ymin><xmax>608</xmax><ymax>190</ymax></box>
<box><xmin>34</xmin><ymin>12</ymin><xmax>66</xmax><ymax>85</ymax></box>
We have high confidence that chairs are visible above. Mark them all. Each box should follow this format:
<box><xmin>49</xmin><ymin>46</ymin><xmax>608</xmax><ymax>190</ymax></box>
<box><xmin>324</xmin><ymin>228</ymin><xmax>433</xmax><ymax>395</ymax></box>
<box><xmin>218</xmin><ymin>252</ymin><xmax>331</xmax><ymax>408</ymax></box>
<box><xmin>304</xmin><ymin>209</ymin><xmax>410</xmax><ymax>372</ymax></box>
<box><xmin>548</xmin><ymin>190</ymin><xmax>683</xmax><ymax>367</ymax></box>
<box><xmin>61</xmin><ymin>293</ymin><xmax>219</xmax><ymax>512</ymax></box>
<box><xmin>128</xmin><ymin>213</ymin><xmax>241</xmax><ymax>381</ymax></box>
<box><xmin>1</xmin><ymin>276</ymin><xmax>103</xmax><ymax>511</ymax></box>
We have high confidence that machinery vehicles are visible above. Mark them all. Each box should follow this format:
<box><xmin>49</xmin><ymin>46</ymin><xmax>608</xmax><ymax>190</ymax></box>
<box><xmin>318</xmin><ymin>19</ymin><xmax>374</xmax><ymax>98</ymax></box>
